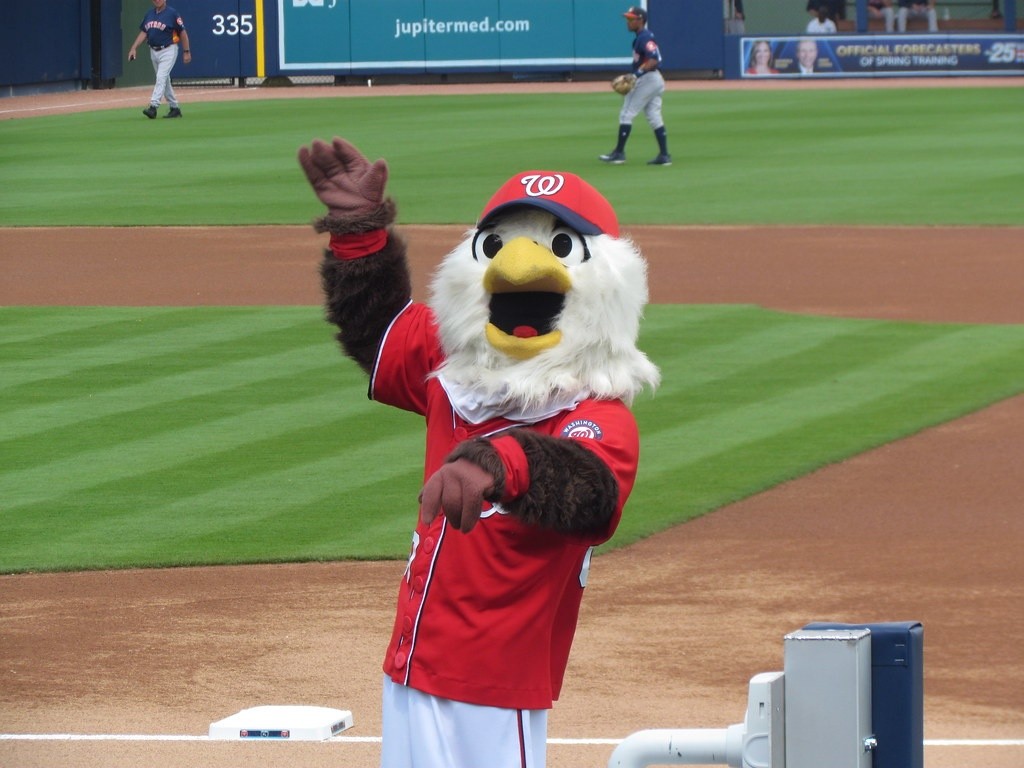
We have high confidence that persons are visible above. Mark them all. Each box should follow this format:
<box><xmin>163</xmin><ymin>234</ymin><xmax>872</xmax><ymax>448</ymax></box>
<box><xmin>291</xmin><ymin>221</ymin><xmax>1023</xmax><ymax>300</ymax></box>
<box><xmin>733</xmin><ymin>0</ymin><xmax>746</xmax><ymax>34</ymax></box>
<box><xmin>783</xmin><ymin>41</ymin><xmax>834</xmax><ymax>73</ymax></box>
<box><xmin>128</xmin><ymin>0</ymin><xmax>192</xmax><ymax>120</ymax></box>
<box><xmin>807</xmin><ymin>0</ymin><xmax>847</xmax><ymax>23</ymax></box>
<box><xmin>867</xmin><ymin>0</ymin><xmax>939</xmax><ymax>33</ymax></box>
<box><xmin>599</xmin><ymin>7</ymin><xmax>672</xmax><ymax>165</ymax></box>
<box><xmin>298</xmin><ymin>140</ymin><xmax>660</xmax><ymax>768</ymax></box>
<box><xmin>807</xmin><ymin>7</ymin><xmax>838</xmax><ymax>35</ymax></box>
<box><xmin>745</xmin><ymin>40</ymin><xmax>779</xmax><ymax>74</ymax></box>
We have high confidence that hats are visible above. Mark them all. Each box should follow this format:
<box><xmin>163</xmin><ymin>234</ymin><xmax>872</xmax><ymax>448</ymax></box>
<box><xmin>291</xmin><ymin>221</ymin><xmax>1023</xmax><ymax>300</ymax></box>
<box><xmin>622</xmin><ymin>6</ymin><xmax>648</xmax><ymax>22</ymax></box>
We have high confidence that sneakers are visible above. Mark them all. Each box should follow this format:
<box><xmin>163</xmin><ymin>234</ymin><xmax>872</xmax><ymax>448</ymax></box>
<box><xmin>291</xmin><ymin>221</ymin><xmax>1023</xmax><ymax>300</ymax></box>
<box><xmin>597</xmin><ymin>153</ymin><xmax>625</xmax><ymax>163</ymax></box>
<box><xmin>143</xmin><ymin>106</ymin><xmax>158</xmax><ymax>119</ymax></box>
<box><xmin>163</xmin><ymin>108</ymin><xmax>181</xmax><ymax>118</ymax></box>
<box><xmin>647</xmin><ymin>152</ymin><xmax>671</xmax><ymax>165</ymax></box>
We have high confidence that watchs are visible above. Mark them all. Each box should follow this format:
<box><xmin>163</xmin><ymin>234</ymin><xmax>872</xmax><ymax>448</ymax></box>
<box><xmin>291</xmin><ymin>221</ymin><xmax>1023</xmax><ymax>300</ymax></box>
<box><xmin>183</xmin><ymin>50</ymin><xmax>190</xmax><ymax>53</ymax></box>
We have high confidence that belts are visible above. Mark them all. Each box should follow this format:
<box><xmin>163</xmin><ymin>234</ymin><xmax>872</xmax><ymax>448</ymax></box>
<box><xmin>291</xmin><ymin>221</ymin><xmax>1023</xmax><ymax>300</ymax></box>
<box><xmin>150</xmin><ymin>44</ymin><xmax>169</xmax><ymax>51</ymax></box>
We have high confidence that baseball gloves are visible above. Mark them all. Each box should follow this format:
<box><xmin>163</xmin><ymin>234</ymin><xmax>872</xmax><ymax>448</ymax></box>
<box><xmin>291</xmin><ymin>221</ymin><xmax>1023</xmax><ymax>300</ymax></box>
<box><xmin>612</xmin><ymin>73</ymin><xmax>636</xmax><ymax>95</ymax></box>
